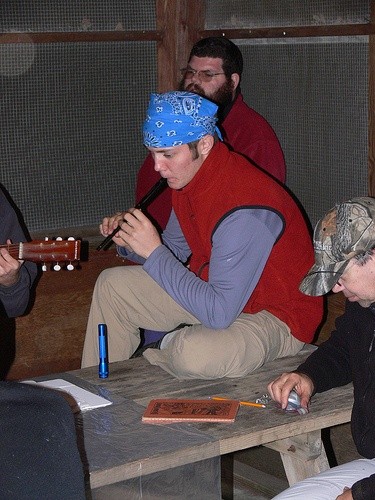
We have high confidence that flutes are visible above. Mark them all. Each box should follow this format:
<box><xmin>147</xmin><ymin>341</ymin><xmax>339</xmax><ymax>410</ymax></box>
<box><xmin>95</xmin><ymin>177</ymin><xmax>168</xmax><ymax>253</ymax></box>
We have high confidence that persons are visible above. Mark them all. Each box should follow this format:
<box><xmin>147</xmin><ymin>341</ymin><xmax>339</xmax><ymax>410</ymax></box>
<box><xmin>0</xmin><ymin>188</ymin><xmax>88</xmax><ymax>500</ymax></box>
<box><xmin>81</xmin><ymin>90</ymin><xmax>323</xmax><ymax>379</ymax></box>
<box><xmin>266</xmin><ymin>196</ymin><xmax>375</xmax><ymax>500</ymax></box>
<box><xmin>135</xmin><ymin>36</ymin><xmax>286</xmax><ymax>232</ymax></box>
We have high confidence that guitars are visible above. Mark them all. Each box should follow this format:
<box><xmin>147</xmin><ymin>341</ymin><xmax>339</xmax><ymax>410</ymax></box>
<box><xmin>0</xmin><ymin>236</ymin><xmax>82</xmax><ymax>271</ymax></box>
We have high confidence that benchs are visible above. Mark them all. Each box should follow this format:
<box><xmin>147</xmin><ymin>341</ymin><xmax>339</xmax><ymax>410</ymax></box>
<box><xmin>17</xmin><ymin>341</ymin><xmax>355</xmax><ymax>488</ymax></box>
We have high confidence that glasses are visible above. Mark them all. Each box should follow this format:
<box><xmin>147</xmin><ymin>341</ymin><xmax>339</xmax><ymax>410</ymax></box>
<box><xmin>183</xmin><ymin>68</ymin><xmax>224</xmax><ymax>81</ymax></box>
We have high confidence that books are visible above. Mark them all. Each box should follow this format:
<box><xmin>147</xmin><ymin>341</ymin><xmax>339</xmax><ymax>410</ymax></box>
<box><xmin>141</xmin><ymin>399</ymin><xmax>240</xmax><ymax>424</ymax></box>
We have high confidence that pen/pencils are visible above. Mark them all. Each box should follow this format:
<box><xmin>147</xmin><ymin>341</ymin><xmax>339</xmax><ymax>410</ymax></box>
<box><xmin>209</xmin><ymin>395</ymin><xmax>266</xmax><ymax>408</ymax></box>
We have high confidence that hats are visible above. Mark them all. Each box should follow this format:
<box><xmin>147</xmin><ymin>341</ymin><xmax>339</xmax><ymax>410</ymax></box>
<box><xmin>298</xmin><ymin>198</ymin><xmax>375</xmax><ymax>296</ymax></box>
